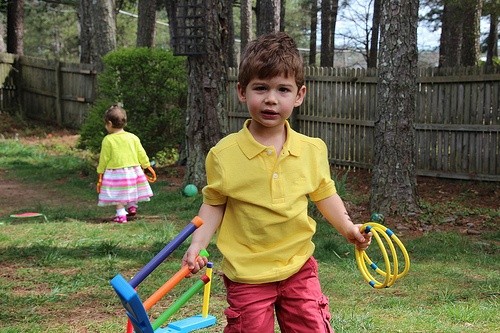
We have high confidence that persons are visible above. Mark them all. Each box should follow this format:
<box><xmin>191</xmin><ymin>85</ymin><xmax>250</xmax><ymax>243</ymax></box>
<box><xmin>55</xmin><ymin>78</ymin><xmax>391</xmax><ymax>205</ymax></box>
<box><xmin>95</xmin><ymin>106</ymin><xmax>155</xmax><ymax>224</ymax></box>
<box><xmin>181</xmin><ymin>31</ymin><xmax>373</xmax><ymax>332</ymax></box>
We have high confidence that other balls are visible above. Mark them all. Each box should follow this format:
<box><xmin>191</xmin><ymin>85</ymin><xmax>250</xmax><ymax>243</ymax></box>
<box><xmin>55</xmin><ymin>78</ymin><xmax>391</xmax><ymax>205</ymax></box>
<box><xmin>185</xmin><ymin>184</ymin><xmax>198</xmax><ymax>196</ymax></box>
<box><xmin>370</xmin><ymin>211</ymin><xmax>386</xmax><ymax>226</ymax></box>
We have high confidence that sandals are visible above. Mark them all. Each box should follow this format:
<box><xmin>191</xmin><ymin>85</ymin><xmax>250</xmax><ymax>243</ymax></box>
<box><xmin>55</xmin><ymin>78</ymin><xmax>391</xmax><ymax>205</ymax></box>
<box><xmin>112</xmin><ymin>216</ymin><xmax>128</xmax><ymax>223</ymax></box>
<box><xmin>126</xmin><ymin>206</ymin><xmax>137</xmax><ymax>215</ymax></box>
